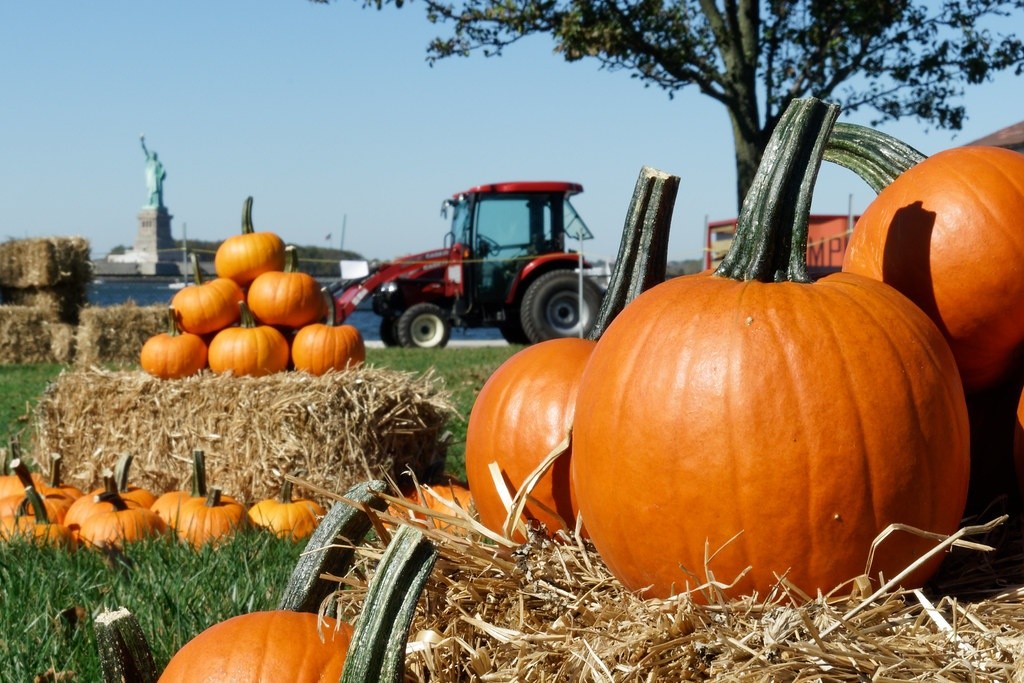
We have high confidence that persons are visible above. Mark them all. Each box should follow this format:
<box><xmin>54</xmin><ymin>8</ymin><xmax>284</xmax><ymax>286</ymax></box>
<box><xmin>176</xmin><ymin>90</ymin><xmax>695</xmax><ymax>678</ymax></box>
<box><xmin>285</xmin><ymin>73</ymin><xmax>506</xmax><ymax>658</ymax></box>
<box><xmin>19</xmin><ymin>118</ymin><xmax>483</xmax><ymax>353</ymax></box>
<box><xmin>141</xmin><ymin>136</ymin><xmax>166</xmax><ymax>207</ymax></box>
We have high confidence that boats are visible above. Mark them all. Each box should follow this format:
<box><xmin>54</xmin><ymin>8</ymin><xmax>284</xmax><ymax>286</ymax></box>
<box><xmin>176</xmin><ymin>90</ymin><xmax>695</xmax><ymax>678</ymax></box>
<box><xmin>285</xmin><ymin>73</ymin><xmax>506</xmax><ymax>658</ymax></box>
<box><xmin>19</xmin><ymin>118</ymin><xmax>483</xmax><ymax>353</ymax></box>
<box><xmin>168</xmin><ymin>278</ymin><xmax>195</xmax><ymax>290</ymax></box>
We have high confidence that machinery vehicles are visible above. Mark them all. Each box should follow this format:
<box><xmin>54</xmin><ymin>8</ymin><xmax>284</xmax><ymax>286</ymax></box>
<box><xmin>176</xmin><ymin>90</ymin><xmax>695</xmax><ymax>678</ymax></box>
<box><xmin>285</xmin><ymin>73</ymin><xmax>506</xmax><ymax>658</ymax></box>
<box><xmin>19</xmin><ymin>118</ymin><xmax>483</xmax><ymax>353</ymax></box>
<box><xmin>321</xmin><ymin>181</ymin><xmax>607</xmax><ymax>349</ymax></box>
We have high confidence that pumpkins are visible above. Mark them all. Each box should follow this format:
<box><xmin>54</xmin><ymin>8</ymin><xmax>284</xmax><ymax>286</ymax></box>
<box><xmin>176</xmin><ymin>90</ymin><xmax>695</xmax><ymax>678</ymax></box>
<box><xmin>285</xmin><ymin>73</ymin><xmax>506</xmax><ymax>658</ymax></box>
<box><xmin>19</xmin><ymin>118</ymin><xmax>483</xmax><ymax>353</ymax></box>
<box><xmin>0</xmin><ymin>97</ymin><xmax>1024</xmax><ymax>683</ymax></box>
